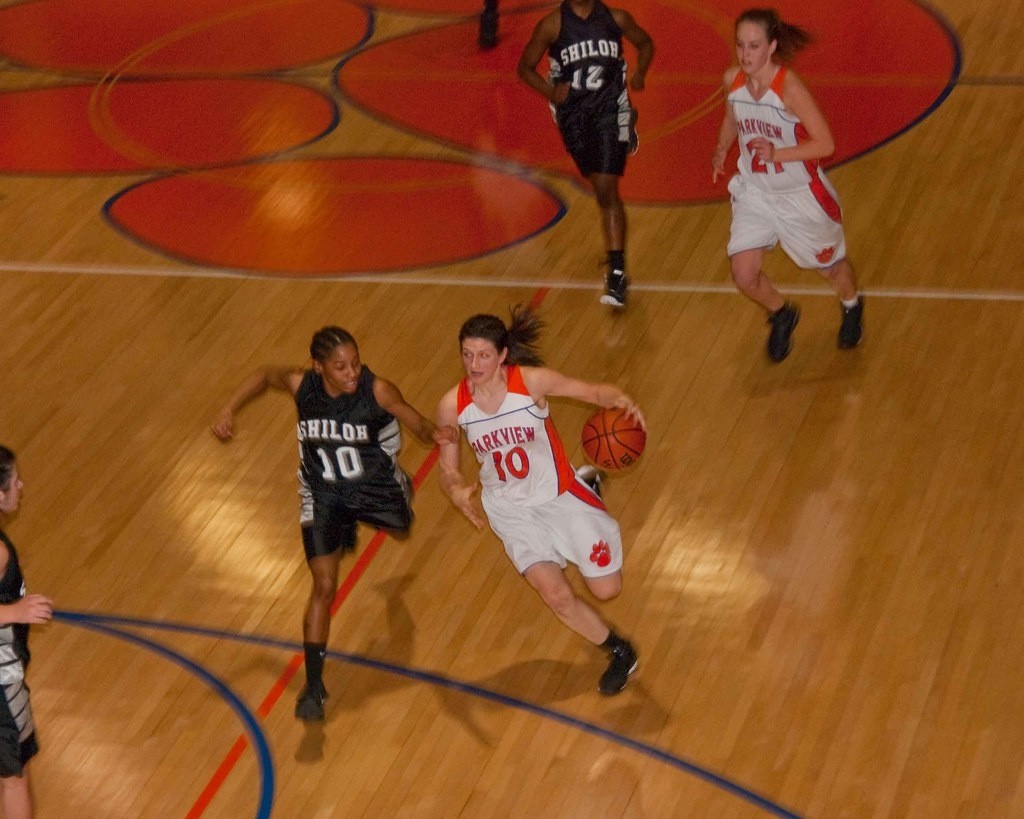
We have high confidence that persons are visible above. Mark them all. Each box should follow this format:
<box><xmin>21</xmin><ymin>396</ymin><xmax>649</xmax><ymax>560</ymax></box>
<box><xmin>516</xmin><ymin>0</ymin><xmax>656</xmax><ymax>312</ymax></box>
<box><xmin>436</xmin><ymin>303</ymin><xmax>647</xmax><ymax>696</ymax></box>
<box><xmin>210</xmin><ymin>324</ymin><xmax>459</xmax><ymax>717</ymax></box>
<box><xmin>0</xmin><ymin>443</ymin><xmax>55</xmax><ymax>819</ymax></box>
<box><xmin>711</xmin><ymin>7</ymin><xmax>865</xmax><ymax>362</ymax></box>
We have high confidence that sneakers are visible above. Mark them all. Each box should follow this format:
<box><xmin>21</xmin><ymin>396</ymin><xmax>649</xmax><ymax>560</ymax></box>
<box><xmin>477</xmin><ymin>13</ymin><xmax>499</xmax><ymax>44</ymax></box>
<box><xmin>599</xmin><ymin>265</ymin><xmax>627</xmax><ymax>307</ymax></box>
<box><xmin>576</xmin><ymin>464</ymin><xmax>609</xmax><ymax>486</ymax></box>
<box><xmin>767</xmin><ymin>300</ymin><xmax>802</xmax><ymax>363</ymax></box>
<box><xmin>596</xmin><ymin>640</ymin><xmax>639</xmax><ymax>695</ymax></box>
<box><xmin>294</xmin><ymin>682</ymin><xmax>328</xmax><ymax>721</ymax></box>
<box><xmin>398</xmin><ymin>464</ymin><xmax>412</xmax><ymax>489</ymax></box>
<box><xmin>838</xmin><ymin>298</ymin><xmax>864</xmax><ymax>350</ymax></box>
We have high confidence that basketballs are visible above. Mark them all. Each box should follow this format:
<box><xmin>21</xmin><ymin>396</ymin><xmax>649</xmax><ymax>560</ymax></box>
<box><xmin>581</xmin><ymin>407</ymin><xmax>647</xmax><ymax>473</ymax></box>
